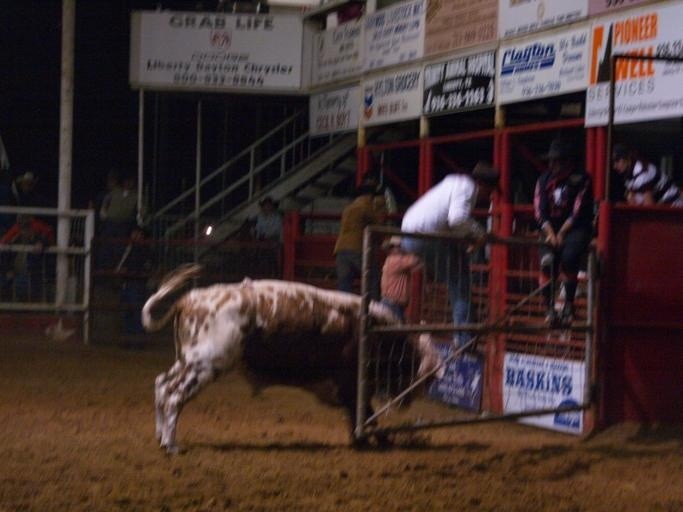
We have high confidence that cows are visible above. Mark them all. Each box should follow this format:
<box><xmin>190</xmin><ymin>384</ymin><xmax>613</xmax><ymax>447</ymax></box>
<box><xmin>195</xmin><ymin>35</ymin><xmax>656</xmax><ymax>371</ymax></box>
<box><xmin>141</xmin><ymin>261</ymin><xmax>449</xmax><ymax>457</ymax></box>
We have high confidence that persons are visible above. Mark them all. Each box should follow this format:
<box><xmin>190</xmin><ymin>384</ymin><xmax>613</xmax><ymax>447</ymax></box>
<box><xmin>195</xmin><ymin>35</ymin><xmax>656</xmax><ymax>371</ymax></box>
<box><xmin>611</xmin><ymin>141</ymin><xmax>683</xmax><ymax>207</ymax></box>
<box><xmin>249</xmin><ymin>196</ymin><xmax>283</xmax><ymax>278</ymax></box>
<box><xmin>332</xmin><ymin>171</ymin><xmax>501</xmax><ymax>359</ymax></box>
<box><xmin>534</xmin><ymin>140</ymin><xmax>593</xmax><ymax>329</ymax></box>
<box><xmin>99</xmin><ymin>176</ymin><xmax>137</xmax><ymax>231</ymax></box>
<box><xmin>0</xmin><ymin>172</ymin><xmax>53</xmax><ymax>286</ymax></box>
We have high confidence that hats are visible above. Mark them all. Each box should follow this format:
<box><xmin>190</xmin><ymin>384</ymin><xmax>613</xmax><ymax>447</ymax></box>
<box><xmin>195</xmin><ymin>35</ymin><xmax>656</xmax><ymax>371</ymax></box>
<box><xmin>15</xmin><ymin>171</ymin><xmax>40</xmax><ymax>184</ymax></box>
<box><xmin>471</xmin><ymin>160</ymin><xmax>502</xmax><ymax>193</ymax></box>
<box><xmin>259</xmin><ymin>196</ymin><xmax>280</xmax><ymax>208</ymax></box>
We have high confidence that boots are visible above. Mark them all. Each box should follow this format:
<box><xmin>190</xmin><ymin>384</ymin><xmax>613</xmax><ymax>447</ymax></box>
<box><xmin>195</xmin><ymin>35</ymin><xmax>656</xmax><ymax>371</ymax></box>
<box><xmin>540</xmin><ymin>280</ymin><xmax>578</xmax><ymax>327</ymax></box>
